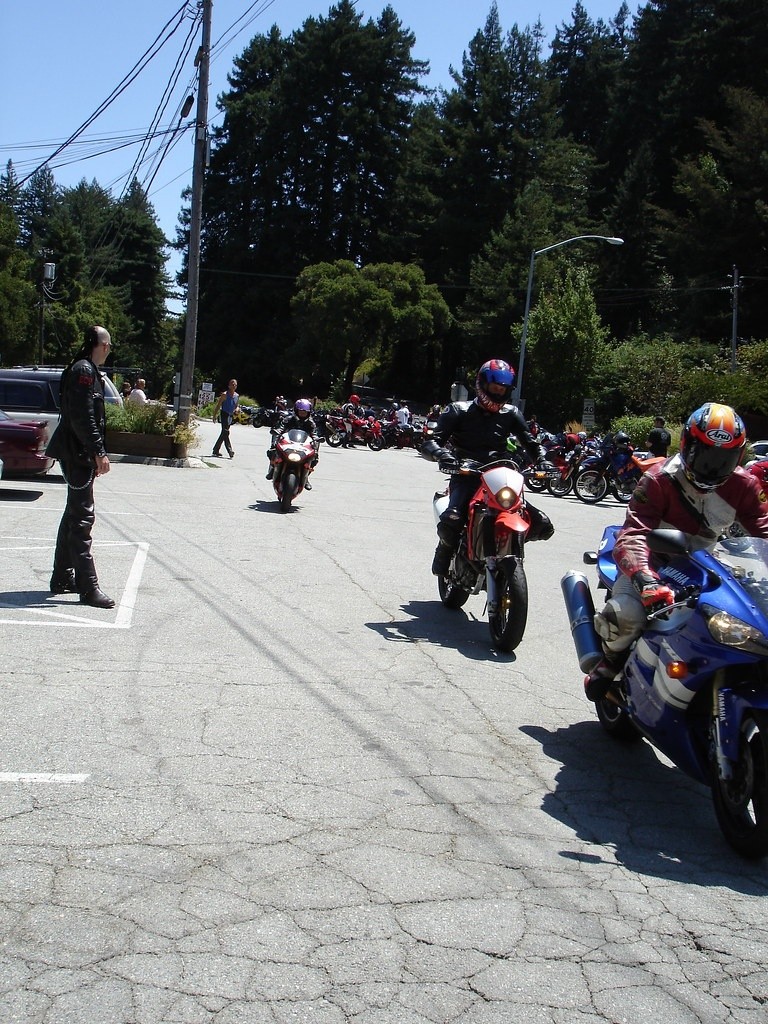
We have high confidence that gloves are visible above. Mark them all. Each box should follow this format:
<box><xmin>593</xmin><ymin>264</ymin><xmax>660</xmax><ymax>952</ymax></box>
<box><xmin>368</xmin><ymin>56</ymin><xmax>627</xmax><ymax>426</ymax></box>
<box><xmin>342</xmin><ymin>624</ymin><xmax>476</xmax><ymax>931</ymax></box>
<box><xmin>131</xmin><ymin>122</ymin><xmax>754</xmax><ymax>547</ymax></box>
<box><xmin>433</xmin><ymin>449</ymin><xmax>458</xmax><ymax>474</ymax></box>
<box><xmin>631</xmin><ymin>569</ymin><xmax>675</xmax><ymax>621</ymax></box>
<box><xmin>535</xmin><ymin>458</ymin><xmax>556</xmax><ymax>472</ymax></box>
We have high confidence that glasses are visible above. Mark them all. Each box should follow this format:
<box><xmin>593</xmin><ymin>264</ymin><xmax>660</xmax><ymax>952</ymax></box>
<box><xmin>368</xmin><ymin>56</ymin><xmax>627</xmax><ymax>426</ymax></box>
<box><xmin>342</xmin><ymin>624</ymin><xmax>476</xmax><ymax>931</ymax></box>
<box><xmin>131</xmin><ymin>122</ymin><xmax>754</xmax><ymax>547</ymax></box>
<box><xmin>298</xmin><ymin>411</ymin><xmax>309</xmax><ymax>415</ymax></box>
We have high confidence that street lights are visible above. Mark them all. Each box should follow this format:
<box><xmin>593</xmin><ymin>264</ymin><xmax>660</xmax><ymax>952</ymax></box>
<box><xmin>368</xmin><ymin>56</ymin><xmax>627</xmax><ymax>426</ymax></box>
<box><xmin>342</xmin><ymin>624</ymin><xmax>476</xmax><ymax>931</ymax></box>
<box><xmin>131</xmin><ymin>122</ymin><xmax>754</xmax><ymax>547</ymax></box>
<box><xmin>511</xmin><ymin>234</ymin><xmax>624</xmax><ymax>407</ymax></box>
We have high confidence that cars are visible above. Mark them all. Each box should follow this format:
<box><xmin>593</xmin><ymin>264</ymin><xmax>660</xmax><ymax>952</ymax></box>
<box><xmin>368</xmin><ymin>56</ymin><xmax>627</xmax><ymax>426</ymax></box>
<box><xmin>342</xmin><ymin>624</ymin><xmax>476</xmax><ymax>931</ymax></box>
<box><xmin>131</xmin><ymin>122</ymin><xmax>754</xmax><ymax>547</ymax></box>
<box><xmin>0</xmin><ymin>412</ymin><xmax>56</xmax><ymax>477</ymax></box>
<box><xmin>0</xmin><ymin>367</ymin><xmax>125</xmax><ymax>450</ymax></box>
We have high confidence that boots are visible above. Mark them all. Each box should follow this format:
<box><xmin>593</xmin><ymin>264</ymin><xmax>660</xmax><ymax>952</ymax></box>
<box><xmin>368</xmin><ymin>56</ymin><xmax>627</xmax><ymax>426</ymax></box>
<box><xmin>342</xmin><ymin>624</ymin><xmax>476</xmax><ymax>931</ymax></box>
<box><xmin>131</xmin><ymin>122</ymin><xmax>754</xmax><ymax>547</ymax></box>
<box><xmin>342</xmin><ymin>433</ymin><xmax>351</xmax><ymax>449</ymax></box>
<box><xmin>584</xmin><ymin>641</ymin><xmax>631</xmax><ymax>702</ymax></box>
<box><xmin>348</xmin><ymin>443</ymin><xmax>357</xmax><ymax>449</ymax></box>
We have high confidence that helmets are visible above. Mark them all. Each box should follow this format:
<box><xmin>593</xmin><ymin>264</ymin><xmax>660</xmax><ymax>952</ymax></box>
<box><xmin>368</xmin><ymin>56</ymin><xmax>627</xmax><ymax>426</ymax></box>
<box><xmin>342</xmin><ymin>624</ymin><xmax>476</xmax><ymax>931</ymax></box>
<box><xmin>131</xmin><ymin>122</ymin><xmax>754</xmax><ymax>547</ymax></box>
<box><xmin>615</xmin><ymin>433</ymin><xmax>630</xmax><ymax>448</ymax></box>
<box><xmin>577</xmin><ymin>431</ymin><xmax>586</xmax><ymax>441</ymax></box>
<box><xmin>391</xmin><ymin>403</ymin><xmax>398</xmax><ymax>411</ymax></box>
<box><xmin>679</xmin><ymin>403</ymin><xmax>746</xmax><ymax>494</ymax></box>
<box><xmin>476</xmin><ymin>359</ymin><xmax>515</xmax><ymax>412</ymax></box>
<box><xmin>349</xmin><ymin>395</ymin><xmax>360</xmax><ymax>406</ymax></box>
<box><xmin>294</xmin><ymin>399</ymin><xmax>312</xmax><ymax>422</ymax></box>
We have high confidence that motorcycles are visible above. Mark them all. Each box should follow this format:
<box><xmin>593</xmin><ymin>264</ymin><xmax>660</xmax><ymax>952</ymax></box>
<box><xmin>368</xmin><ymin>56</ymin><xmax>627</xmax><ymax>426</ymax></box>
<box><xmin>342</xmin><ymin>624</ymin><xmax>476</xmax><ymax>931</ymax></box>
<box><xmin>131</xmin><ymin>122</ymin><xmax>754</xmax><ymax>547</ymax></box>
<box><xmin>560</xmin><ymin>524</ymin><xmax>768</xmax><ymax>866</ymax></box>
<box><xmin>267</xmin><ymin>427</ymin><xmax>325</xmax><ymax>512</ymax></box>
<box><xmin>229</xmin><ymin>405</ymin><xmax>768</xmax><ymax>553</ymax></box>
<box><xmin>432</xmin><ymin>446</ymin><xmax>564</xmax><ymax>652</ymax></box>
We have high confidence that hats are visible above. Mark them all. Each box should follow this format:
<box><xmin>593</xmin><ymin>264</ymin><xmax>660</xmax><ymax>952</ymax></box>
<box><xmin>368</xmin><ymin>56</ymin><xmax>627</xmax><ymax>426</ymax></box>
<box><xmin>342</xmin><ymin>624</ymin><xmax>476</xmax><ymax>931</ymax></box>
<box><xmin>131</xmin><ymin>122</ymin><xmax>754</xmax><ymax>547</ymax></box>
<box><xmin>653</xmin><ymin>416</ymin><xmax>665</xmax><ymax>424</ymax></box>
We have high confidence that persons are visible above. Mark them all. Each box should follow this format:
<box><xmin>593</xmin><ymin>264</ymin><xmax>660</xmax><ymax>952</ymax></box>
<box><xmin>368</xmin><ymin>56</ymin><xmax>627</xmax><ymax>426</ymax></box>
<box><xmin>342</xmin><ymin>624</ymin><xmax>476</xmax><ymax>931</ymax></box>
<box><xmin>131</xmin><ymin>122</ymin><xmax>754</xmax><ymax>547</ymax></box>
<box><xmin>584</xmin><ymin>402</ymin><xmax>768</xmax><ymax>701</ymax></box>
<box><xmin>421</xmin><ymin>359</ymin><xmax>554</xmax><ymax>577</ymax></box>
<box><xmin>276</xmin><ymin>395</ymin><xmax>287</xmax><ymax>411</ymax></box>
<box><xmin>44</xmin><ymin>326</ymin><xmax>115</xmax><ymax>608</ymax></box>
<box><xmin>266</xmin><ymin>399</ymin><xmax>319</xmax><ymax>491</ymax></box>
<box><xmin>392</xmin><ymin>400</ymin><xmax>412</xmax><ymax>449</ymax></box>
<box><xmin>212</xmin><ymin>379</ymin><xmax>239</xmax><ymax>457</ymax></box>
<box><xmin>526</xmin><ymin>414</ymin><xmax>540</xmax><ymax>437</ymax></box>
<box><xmin>646</xmin><ymin>417</ymin><xmax>671</xmax><ymax>458</ymax></box>
<box><xmin>427</xmin><ymin>404</ymin><xmax>441</xmax><ymax>422</ymax></box>
<box><xmin>342</xmin><ymin>394</ymin><xmax>375</xmax><ymax>448</ymax></box>
<box><xmin>123</xmin><ymin>379</ymin><xmax>147</xmax><ymax>405</ymax></box>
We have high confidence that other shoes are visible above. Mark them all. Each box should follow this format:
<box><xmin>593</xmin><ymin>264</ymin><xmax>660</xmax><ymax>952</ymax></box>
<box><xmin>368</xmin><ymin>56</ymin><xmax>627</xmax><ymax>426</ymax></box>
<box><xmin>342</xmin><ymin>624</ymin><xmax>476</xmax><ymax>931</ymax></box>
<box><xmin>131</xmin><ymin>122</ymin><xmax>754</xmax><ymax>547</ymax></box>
<box><xmin>432</xmin><ymin>542</ymin><xmax>456</xmax><ymax>576</ymax></box>
<box><xmin>266</xmin><ymin>468</ymin><xmax>273</xmax><ymax>480</ymax></box>
<box><xmin>305</xmin><ymin>481</ymin><xmax>312</xmax><ymax>490</ymax></box>
<box><xmin>229</xmin><ymin>451</ymin><xmax>234</xmax><ymax>457</ymax></box>
<box><xmin>394</xmin><ymin>446</ymin><xmax>403</xmax><ymax>449</ymax></box>
<box><xmin>212</xmin><ymin>451</ymin><xmax>222</xmax><ymax>456</ymax></box>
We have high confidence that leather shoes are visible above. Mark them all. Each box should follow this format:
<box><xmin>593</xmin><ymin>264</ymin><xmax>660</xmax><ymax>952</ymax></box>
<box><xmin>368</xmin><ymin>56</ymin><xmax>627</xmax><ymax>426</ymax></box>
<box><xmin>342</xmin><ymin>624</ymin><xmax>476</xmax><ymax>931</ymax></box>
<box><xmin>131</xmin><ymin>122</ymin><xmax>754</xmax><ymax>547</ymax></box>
<box><xmin>50</xmin><ymin>573</ymin><xmax>78</xmax><ymax>594</ymax></box>
<box><xmin>80</xmin><ymin>587</ymin><xmax>115</xmax><ymax>607</ymax></box>
